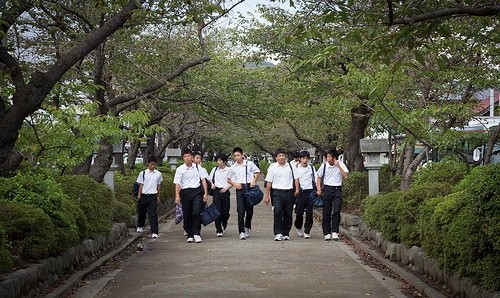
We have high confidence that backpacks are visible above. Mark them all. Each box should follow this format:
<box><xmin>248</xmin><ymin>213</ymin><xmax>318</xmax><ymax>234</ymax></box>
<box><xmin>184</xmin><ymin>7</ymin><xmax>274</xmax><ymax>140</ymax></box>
<box><xmin>244</xmin><ymin>185</ymin><xmax>264</xmax><ymax>206</ymax></box>
<box><xmin>133</xmin><ymin>169</ymin><xmax>145</xmax><ymax>198</ymax></box>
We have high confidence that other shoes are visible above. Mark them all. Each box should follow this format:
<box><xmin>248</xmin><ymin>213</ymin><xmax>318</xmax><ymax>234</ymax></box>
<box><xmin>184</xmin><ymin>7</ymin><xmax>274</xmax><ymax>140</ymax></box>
<box><xmin>216</xmin><ymin>232</ymin><xmax>223</xmax><ymax>237</ymax></box>
<box><xmin>297</xmin><ymin>228</ymin><xmax>303</xmax><ymax>237</ymax></box>
<box><xmin>194</xmin><ymin>234</ymin><xmax>202</xmax><ymax>242</ymax></box>
<box><xmin>187</xmin><ymin>238</ymin><xmax>194</xmax><ymax>243</ymax></box>
<box><xmin>183</xmin><ymin>229</ymin><xmax>188</xmax><ymax>236</ymax></box>
<box><xmin>137</xmin><ymin>226</ymin><xmax>144</xmax><ymax>233</ymax></box>
<box><xmin>303</xmin><ymin>231</ymin><xmax>311</xmax><ymax>239</ymax></box>
<box><xmin>152</xmin><ymin>233</ymin><xmax>158</xmax><ymax>239</ymax></box>
<box><xmin>222</xmin><ymin>225</ymin><xmax>227</xmax><ymax>233</ymax></box>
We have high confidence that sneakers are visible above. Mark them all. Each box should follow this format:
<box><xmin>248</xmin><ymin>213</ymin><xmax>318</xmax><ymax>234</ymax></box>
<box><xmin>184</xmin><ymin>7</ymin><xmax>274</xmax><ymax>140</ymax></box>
<box><xmin>284</xmin><ymin>235</ymin><xmax>289</xmax><ymax>240</ymax></box>
<box><xmin>274</xmin><ymin>233</ymin><xmax>283</xmax><ymax>241</ymax></box>
<box><xmin>324</xmin><ymin>234</ymin><xmax>331</xmax><ymax>240</ymax></box>
<box><xmin>239</xmin><ymin>232</ymin><xmax>245</xmax><ymax>239</ymax></box>
<box><xmin>244</xmin><ymin>226</ymin><xmax>250</xmax><ymax>236</ymax></box>
<box><xmin>332</xmin><ymin>232</ymin><xmax>339</xmax><ymax>240</ymax></box>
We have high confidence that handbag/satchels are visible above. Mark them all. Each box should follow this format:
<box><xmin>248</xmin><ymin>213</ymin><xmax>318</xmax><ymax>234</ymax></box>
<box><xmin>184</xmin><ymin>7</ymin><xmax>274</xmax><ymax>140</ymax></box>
<box><xmin>196</xmin><ymin>163</ymin><xmax>211</xmax><ymax>194</ymax></box>
<box><xmin>309</xmin><ymin>190</ymin><xmax>324</xmax><ymax>208</ymax></box>
<box><xmin>209</xmin><ymin>182</ymin><xmax>215</xmax><ymax>196</ymax></box>
<box><xmin>200</xmin><ymin>205</ymin><xmax>221</xmax><ymax>226</ymax></box>
<box><xmin>294</xmin><ymin>188</ymin><xmax>303</xmax><ymax>204</ymax></box>
<box><xmin>293</xmin><ymin>179</ymin><xmax>303</xmax><ymax>197</ymax></box>
<box><xmin>175</xmin><ymin>199</ymin><xmax>183</xmax><ymax>224</ymax></box>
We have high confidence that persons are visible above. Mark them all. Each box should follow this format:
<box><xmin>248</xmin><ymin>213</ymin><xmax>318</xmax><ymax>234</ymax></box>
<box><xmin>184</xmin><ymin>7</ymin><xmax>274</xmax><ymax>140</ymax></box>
<box><xmin>173</xmin><ymin>148</ymin><xmax>208</xmax><ymax>243</ymax></box>
<box><xmin>264</xmin><ymin>151</ymin><xmax>306</xmax><ymax>215</ymax></box>
<box><xmin>264</xmin><ymin>148</ymin><xmax>299</xmax><ymax>241</ymax></box>
<box><xmin>316</xmin><ymin>149</ymin><xmax>349</xmax><ymax>241</ymax></box>
<box><xmin>208</xmin><ymin>153</ymin><xmax>235</xmax><ymax>237</ymax></box>
<box><xmin>136</xmin><ymin>156</ymin><xmax>163</xmax><ymax>238</ymax></box>
<box><xmin>293</xmin><ymin>151</ymin><xmax>318</xmax><ymax>239</ymax></box>
<box><xmin>227</xmin><ymin>152</ymin><xmax>260</xmax><ymax>170</ymax></box>
<box><xmin>227</xmin><ymin>147</ymin><xmax>261</xmax><ymax>240</ymax></box>
